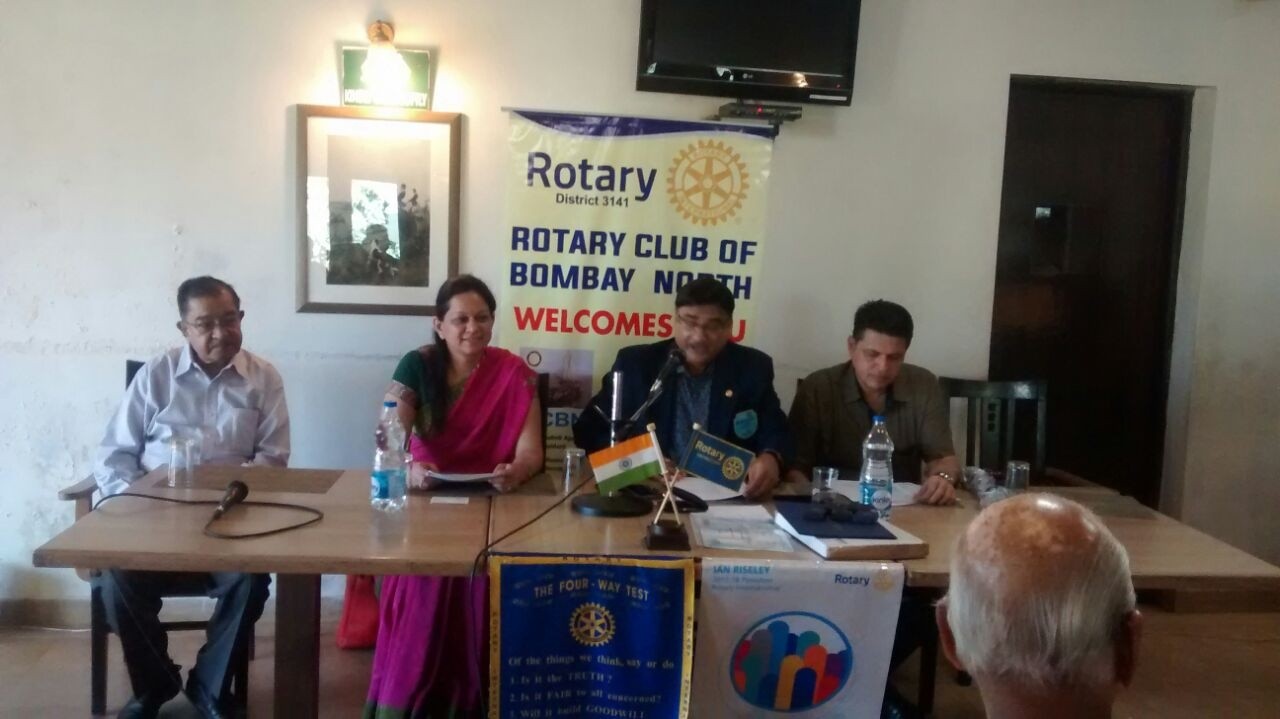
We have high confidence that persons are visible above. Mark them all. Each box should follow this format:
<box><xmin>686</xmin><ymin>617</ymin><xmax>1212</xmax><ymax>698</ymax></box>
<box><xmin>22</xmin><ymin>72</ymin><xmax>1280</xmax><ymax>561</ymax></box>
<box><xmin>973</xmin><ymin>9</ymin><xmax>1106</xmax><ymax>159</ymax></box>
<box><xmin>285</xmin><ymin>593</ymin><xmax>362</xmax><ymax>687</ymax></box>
<box><xmin>785</xmin><ymin>298</ymin><xmax>962</xmax><ymax>716</ymax></box>
<box><xmin>933</xmin><ymin>490</ymin><xmax>1142</xmax><ymax>718</ymax></box>
<box><xmin>94</xmin><ymin>275</ymin><xmax>292</xmax><ymax>719</ymax></box>
<box><xmin>361</xmin><ymin>274</ymin><xmax>544</xmax><ymax>719</ymax></box>
<box><xmin>575</xmin><ymin>280</ymin><xmax>788</xmax><ymax>500</ymax></box>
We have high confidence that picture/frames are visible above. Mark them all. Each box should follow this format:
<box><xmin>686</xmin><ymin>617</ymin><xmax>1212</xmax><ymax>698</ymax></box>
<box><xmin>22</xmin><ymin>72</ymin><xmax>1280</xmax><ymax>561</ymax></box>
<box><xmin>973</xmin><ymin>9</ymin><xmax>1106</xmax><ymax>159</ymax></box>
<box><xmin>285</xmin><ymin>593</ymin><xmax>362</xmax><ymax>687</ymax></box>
<box><xmin>294</xmin><ymin>102</ymin><xmax>464</xmax><ymax>317</ymax></box>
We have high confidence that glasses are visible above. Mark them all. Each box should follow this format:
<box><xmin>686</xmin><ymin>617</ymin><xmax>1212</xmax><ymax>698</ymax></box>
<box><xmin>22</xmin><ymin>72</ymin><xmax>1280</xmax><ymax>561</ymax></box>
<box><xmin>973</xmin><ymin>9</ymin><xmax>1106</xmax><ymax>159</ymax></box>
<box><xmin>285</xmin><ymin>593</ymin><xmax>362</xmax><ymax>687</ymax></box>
<box><xmin>675</xmin><ymin>311</ymin><xmax>731</xmax><ymax>336</ymax></box>
<box><xmin>183</xmin><ymin>310</ymin><xmax>240</xmax><ymax>334</ymax></box>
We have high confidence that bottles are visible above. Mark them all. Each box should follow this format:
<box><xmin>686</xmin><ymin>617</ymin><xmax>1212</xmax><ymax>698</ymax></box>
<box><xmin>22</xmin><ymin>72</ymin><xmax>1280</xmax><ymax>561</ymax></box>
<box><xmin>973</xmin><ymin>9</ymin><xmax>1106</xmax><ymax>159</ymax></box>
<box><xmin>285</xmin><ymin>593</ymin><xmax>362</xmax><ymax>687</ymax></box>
<box><xmin>372</xmin><ymin>401</ymin><xmax>408</xmax><ymax>512</ymax></box>
<box><xmin>858</xmin><ymin>415</ymin><xmax>895</xmax><ymax>519</ymax></box>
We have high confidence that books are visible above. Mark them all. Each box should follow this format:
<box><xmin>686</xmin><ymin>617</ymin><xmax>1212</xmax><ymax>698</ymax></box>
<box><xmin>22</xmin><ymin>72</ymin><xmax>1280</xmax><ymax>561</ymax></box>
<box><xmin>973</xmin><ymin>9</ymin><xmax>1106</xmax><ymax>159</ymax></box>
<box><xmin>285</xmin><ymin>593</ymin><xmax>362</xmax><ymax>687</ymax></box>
<box><xmin>775</xmin><ymin>510</ymin><xmax>929</xmax><ymax>561</ymax></box>
<box><xmin>424</xmin><ymin>468</ymin><xmax>495</xmax><ymax>483</ymax></box>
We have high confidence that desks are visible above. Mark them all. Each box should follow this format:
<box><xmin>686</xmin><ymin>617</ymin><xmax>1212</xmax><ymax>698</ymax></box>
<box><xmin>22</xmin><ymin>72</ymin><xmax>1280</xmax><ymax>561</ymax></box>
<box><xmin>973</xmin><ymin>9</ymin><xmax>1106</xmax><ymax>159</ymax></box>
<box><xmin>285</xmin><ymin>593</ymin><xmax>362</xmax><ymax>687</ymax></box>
<box><xmin>36</xmin><ymin>464</ymin><xmax>491</xmax><ymax>719</ymax></box>
<box><xmin>811</xmin><ymin>484</ymin><xmax>1279</xmax><ymax>719</ymax></box>
<box><xmin>487</xmin><ymin>481</ymin><xmax>905</xmax><ymax>719</ymax></box>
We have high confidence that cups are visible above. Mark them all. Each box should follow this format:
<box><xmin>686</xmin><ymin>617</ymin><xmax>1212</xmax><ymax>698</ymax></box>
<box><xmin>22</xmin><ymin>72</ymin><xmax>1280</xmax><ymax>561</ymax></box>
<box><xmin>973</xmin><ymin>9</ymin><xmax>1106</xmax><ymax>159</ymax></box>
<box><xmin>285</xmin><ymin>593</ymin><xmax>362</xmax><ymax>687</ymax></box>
<box><xmin>168</xmin><ymin>439</ymin><xmax>196</xmax><ymax>489</ymax></box>
<box><xmin>1006</xmin><ymin>460</ymin><xmax>1031</xmax><ymax>497</ymax></box>
<box><xmin>559</xmin><ymin>447</ymin><xmax>589</xmax><ymax>498</ymax></box>
<box><xmin>811</xmin><ymin>468</ymin><xmax>839</xmax><ymax>497</ymax></box>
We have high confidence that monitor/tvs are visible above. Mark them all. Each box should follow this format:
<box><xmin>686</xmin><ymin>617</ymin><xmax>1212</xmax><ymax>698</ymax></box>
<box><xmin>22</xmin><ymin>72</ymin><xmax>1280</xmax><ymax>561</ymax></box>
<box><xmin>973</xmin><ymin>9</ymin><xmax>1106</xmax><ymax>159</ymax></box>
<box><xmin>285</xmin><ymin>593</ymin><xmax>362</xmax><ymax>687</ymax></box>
<box><xmin>636</xmin><ymin>0</ymin><xmax>862</xmax><ymax>107</ymax></box>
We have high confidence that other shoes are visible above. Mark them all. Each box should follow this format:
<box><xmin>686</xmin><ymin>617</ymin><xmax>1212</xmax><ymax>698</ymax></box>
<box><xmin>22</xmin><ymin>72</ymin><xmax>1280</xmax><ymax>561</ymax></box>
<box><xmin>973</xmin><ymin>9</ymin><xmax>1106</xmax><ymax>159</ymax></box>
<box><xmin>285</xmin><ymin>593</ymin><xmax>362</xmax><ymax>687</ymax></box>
<box><xmin>118</xmin><ymin>664</ymin><xmax>182</xmax><ymax>719</ymax></box>
<box><xmin>185</xmin><ymin>669</ymin><xmax>234</xmax><ymax>719</ymax></box>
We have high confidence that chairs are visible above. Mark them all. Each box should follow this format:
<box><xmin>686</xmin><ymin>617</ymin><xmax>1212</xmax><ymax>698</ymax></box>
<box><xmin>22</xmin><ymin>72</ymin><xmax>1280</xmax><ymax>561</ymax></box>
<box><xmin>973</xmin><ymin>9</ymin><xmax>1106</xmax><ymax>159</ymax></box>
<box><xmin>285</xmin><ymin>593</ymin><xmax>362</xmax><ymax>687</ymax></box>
<box><xmin>59</xmin><ymin>358</ymin><xmax>257</xmax><ymax>717</ymax></box>
<box><xmin>939</xmin><ymin>374</ymin><xmax>1047</xmax><ymax>484</ymax></box>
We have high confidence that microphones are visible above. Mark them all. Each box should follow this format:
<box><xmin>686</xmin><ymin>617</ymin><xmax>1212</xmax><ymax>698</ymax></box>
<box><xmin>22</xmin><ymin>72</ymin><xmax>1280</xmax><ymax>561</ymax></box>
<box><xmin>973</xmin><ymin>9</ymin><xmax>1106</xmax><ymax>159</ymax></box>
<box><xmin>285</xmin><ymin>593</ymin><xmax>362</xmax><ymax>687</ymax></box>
<box><xmin>211</xmin><ymin>480</ymin><xmax>248</xmax><ymax>522</ymax></box>
<box><xmin>648</xmin><ymin>349</ymin><xmax>684</xmax><ymax>401</ymax></box>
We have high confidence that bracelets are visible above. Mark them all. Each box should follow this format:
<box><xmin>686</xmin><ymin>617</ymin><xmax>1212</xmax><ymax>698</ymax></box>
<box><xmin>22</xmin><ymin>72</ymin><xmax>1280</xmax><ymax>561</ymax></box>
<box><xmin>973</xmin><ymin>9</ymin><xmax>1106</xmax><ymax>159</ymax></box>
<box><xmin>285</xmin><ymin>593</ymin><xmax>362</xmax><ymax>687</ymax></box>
<box><xmin>934</xmin><ymin>470</ymin><xmax>955</xmax><ymax>484</ymax></box>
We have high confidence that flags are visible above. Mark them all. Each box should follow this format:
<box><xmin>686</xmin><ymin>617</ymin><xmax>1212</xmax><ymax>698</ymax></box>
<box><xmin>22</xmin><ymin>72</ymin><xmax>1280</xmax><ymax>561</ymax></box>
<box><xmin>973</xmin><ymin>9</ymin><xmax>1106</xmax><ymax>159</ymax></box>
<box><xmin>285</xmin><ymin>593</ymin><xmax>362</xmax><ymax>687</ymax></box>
<box><xmin>677</xmin><ymin>422</ymin><xmax>756</xmax><ymax>493</ymax></box>
<box><xmin>588</xmin><ymin>429</ymin><xmax>664</xmax><ymax>497</ymax></box>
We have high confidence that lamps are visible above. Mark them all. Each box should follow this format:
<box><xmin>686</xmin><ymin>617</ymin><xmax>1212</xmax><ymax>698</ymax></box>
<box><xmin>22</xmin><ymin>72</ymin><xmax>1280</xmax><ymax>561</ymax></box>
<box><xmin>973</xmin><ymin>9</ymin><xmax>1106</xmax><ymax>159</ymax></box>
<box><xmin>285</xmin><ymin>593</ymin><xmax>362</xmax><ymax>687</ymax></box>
<box><xmin>362</xmin><ymin>23</ymin><xmax>409</xmax><ymax>86</ymax></box>
